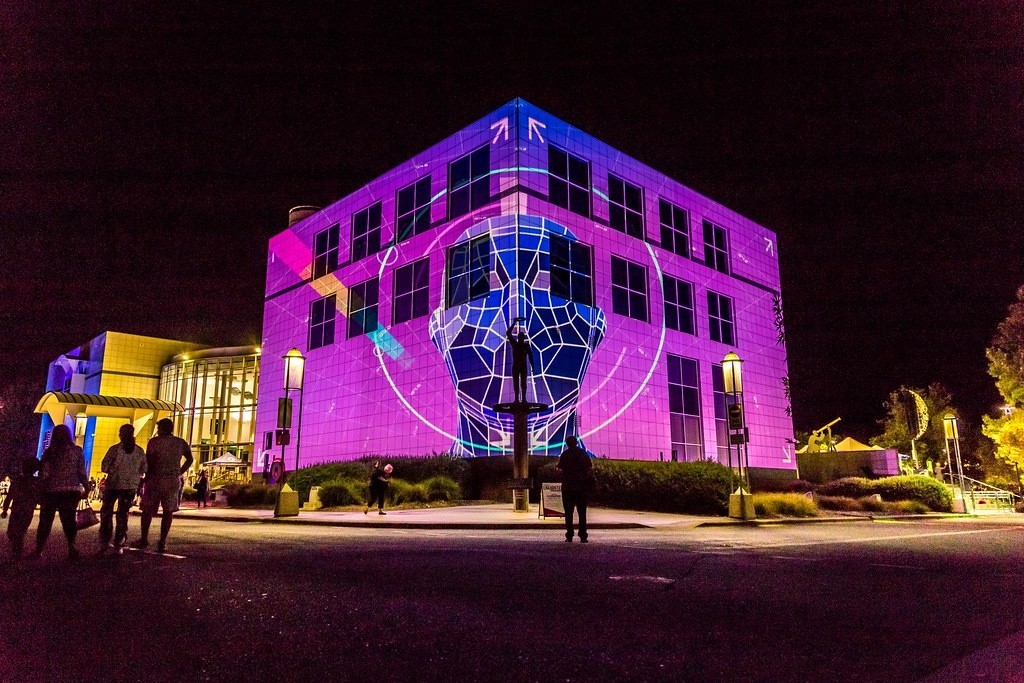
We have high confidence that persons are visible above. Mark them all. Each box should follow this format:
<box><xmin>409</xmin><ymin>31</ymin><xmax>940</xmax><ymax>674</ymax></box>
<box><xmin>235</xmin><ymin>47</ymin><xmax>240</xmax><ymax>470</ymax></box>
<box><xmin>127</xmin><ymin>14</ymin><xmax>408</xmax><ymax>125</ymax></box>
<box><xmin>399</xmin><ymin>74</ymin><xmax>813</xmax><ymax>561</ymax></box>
<box><xmin>933</xmin><ymin>460</ymin><xmax>947</xmax><ymax>480</ymax></box>
<box><xmin>364</xmin><ymin>460</ymin><xmax>391</xmax><ymax>515</ymax></box>
<box><xmin>1014</xmin><ymin>493</ymin><xmax>1024</xmax><ymax>513</ymax></box>
<box><xmin>193</xmin><ymin>469</ymin><xmax>208</xmax><ymax>508</ymax></box>
<box><xmin>0</xmin><ymin>457</ymin><xmax>42</xmax><ymax>555</ymax></box>
<box><xmin>129</xmin><ymin>418</ymin><xmax>194</xmax><ymax>553</ymax></box>
<box><xmin>554</xmin><ymin>436</ymin><xmax>598</xmax><ymax>543</ymax></box>
<box><xmin>32</xmin><ymin>423</ymin><xmax>88</xmax><ymax>561</ymax></box>
<box><xmin>97</xmin><ymin>474</ymin><xmax>107</xmax><ymax>501</ymax></box>
<box><xmin>88</xmin><ymin>477</ymin><xmax>97</xmax><ymax>503</ymax></box>
<box><xmin>506</xmin><ymin>316</ymin><xmax>534</xmax><ymax>403</ymax></box>
<box><xmin>98</xmin><ymin>424</ymin><xmax>148</xmax><ymax>553</ymax></box>
<box><xmin>0</xmin><ymin>476</ymin><xmax>11</xmax><ymax>509</ymax></box>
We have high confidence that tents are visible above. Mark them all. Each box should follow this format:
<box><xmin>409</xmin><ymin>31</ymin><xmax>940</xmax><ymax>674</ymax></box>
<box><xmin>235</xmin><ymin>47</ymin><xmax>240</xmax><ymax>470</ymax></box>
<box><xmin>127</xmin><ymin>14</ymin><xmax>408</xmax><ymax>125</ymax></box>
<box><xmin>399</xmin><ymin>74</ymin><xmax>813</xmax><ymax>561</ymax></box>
<box><xmin>203</xmin><ymin>451</ymin><xmax>249</xmax><ymax>482</ymax></box>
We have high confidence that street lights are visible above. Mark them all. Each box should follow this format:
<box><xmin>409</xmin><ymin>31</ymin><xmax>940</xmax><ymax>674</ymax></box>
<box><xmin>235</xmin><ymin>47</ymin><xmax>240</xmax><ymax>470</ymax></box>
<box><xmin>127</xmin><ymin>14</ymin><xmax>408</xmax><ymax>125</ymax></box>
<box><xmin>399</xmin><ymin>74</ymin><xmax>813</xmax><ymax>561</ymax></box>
<box><xmin>274</xmin><ymin>348</ymin><xmax>307</xmax><ymax>516</ymax></box>
<box><xmin>1000</xmin><ymin>407</ymin><xmax>1023</xmax><ymax>509</ymax></box>
<box><xmin>941</xmin><ymin>411</ymin><xmax>967</xmax><ymax>513</ymax></box>
<box><xmin>720</xmin><ymin>349</ymin><xmax>756</xmax><ymax>520</ymax></box>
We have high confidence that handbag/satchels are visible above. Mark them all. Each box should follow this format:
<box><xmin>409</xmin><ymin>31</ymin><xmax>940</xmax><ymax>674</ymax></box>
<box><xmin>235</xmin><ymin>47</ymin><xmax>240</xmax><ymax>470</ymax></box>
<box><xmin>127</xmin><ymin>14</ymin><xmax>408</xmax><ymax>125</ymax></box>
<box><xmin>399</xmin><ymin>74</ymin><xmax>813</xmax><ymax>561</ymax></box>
<box><xmin>192</xmin><ymin>482</ymin><xmax>202</xmax><ymax>490</ymax></box>
<box><xmin>74</xmin><ymin>493</ymin><xmax>100</xmax><ymax>530</ymax></box>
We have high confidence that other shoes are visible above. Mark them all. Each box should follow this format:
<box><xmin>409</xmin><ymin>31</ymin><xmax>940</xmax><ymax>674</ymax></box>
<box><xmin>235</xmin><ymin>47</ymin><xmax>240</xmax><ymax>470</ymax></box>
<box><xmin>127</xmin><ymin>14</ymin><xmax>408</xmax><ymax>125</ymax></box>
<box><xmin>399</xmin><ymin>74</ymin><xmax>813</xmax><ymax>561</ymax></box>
<box><xmin>565</xmin><ymin>537</ymin><xmax>573</xmax><ymax>543</ymax></box>
<box><xmin>580</xmin><ymin>538</ymin><xmax>589</xmax><ymax>543</ymax></box>
<box><xmin>67</xmin><ymin>546</ymin><xmax>79</xmax><ymax>560</ymax></box>
<box><xmin>131</xmin><ymin>538</ymin><xmax>149</xmax><ymax>547</ymax></box>
<box><xmin>156</xmin><ymin>542</ymin><xmax>168</xmax><ymax>552</ymax></box>
<box><xmin>32</xmin><ymin>545</ymin><xmax>43</xmax><ymax>556</ymax></box>
<box><xmin>11</xmin><ymin>544</ymin><xmax>22</xmax><ymax>555</ymax></box>
<box><xmin>364</xmin><ymin>506</ymin><xmax>369</xmax><ymax>514</ymax></box>
<box><xmin>379</xmin><ymin>511</ymin><xmax>387</xmax><ymax>515</ymax></box>
